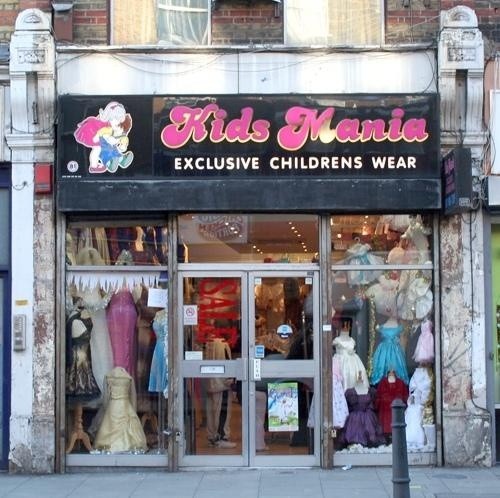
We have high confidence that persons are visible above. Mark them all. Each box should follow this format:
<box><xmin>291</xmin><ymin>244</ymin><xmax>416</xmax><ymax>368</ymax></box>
<box><xmin>63</xmin><ymin>297</ymin><xmax>102</xmax><ymax>403</ymax></box>
<box><xmin>369</xmin><ymin>316</ymin><xmax>411</xmax><ymax>386</ymax></box>
<box><xmin>306</xmin><ymin>321</ymin><xmax>369</xmax><ymax>429</ymax></box>
<box><xmin>373</xmin><ymin>369</ymin><xmax>409</xmax><ymax>434</ymax></box>
<box><xmin>204</xmin><ymin>320</ymin><xmax>237</xmax><ymax>449</ymax></box>
<box><xmin>106</xmin><ymin>287</ymin><xmax>137</xmax><ymax>376</ymax></box>
<box><xmin>148</xmin><ymin>309</ymin><xmax>172</xmax><ymax>393</ymax></box>
<box><xmin>86</xmin><ymin>366</ymin><xmax>149</xmax><ymax>455</ymax></box>
<box><xmin>338</xmin><ymin>372</ymin><xmax>386</xmax><ymax>446</ymax></box>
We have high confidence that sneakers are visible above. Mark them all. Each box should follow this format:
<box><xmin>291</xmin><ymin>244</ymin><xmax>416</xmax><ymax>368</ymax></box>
<box><xmin>208</xmin><ymin>439</ymin><xmax>216</xmax><ymax>447</ymax></box>
<box><xmin>214</xmin><ymin>440</ymin><xmax>236</xmax><ymax>449</ymax></box>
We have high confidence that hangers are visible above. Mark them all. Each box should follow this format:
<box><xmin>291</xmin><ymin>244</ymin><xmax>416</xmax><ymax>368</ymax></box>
<box><xmin>350</xmin><ymin>237</ymin><xmax>364</xmax><ymax>250</ymax></box>
